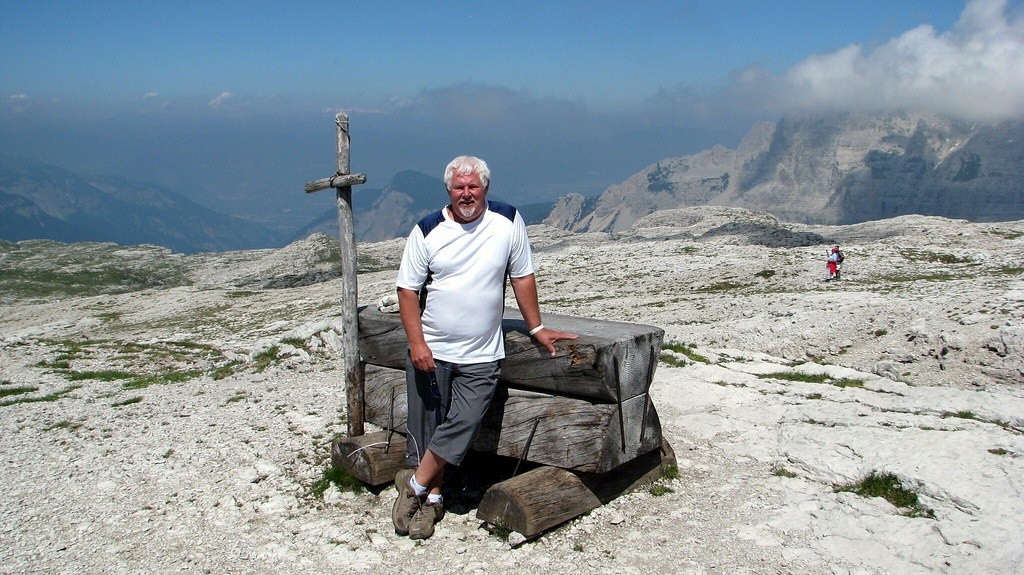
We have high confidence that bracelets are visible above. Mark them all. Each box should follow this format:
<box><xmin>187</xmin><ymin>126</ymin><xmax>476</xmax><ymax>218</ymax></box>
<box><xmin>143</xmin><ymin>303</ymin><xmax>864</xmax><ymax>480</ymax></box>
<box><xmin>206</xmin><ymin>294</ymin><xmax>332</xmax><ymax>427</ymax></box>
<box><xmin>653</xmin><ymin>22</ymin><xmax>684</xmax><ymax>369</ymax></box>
<box><xmin>529</xmin><ymin>324</ymin><xmax>544</xmax><ymax>335</ymax></box>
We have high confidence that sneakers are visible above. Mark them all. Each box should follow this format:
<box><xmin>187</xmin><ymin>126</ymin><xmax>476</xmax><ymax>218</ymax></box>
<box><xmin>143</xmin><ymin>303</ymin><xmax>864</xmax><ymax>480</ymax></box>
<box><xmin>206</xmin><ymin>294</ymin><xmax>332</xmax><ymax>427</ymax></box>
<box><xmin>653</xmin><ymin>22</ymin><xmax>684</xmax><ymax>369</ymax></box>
<box><xmin>392</xmin><ymin>469</ymin><xmax>427</xmax><ymax>535</ymax></box>
<box><xmin>409</xmin><ymin>497</ymin><xmax>444</xmax><ymax>539</ymax></box>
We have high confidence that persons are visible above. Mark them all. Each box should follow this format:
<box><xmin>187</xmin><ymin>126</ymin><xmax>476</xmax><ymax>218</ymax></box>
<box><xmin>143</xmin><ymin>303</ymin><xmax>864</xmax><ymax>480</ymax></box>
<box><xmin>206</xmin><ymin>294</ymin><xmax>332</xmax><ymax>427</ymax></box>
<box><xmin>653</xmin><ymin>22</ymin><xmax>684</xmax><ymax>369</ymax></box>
<box><xmin>828</xmin><ymin>246</ymin><xmax>843</xmax><ymax>279</ymax></box>
<box><xmin>392</xmin><ymin>156</ymin><xmax>578</xmax><ymax>539</ymax></box>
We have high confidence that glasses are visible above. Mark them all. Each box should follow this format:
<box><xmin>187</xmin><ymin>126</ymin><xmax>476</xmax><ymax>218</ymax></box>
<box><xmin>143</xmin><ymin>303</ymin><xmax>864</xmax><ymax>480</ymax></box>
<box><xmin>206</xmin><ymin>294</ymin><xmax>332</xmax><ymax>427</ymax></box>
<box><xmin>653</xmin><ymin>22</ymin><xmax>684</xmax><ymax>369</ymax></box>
<box><xmin>429</xmin><ymin>364</ymin><xmax>457</xmax><ymax>399</ymax></box>
